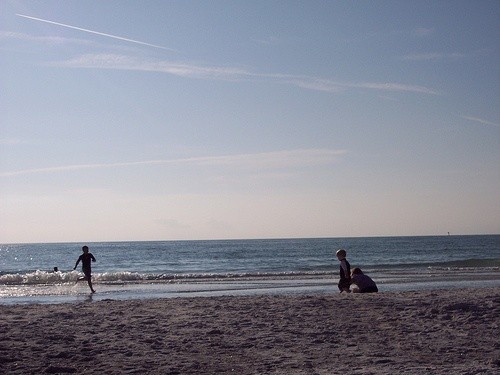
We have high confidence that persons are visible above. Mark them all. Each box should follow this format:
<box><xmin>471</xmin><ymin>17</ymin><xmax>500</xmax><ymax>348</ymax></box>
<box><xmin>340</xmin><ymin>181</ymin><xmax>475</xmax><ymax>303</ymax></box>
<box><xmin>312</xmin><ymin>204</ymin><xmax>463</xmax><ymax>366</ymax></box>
<box><xmin>348</xmin><ymin>268</ymin><xmax>379</xmax><ymax>293</ymax></box>
<box><xmin>73</xmin><ymin>245</ymin><xmax>97</xmax><ymax>294</ymax></box>
<box><xmin>335</xmin><ymin>248</ymin><xmax>353</xmax><ymax>293</ymax></box>
